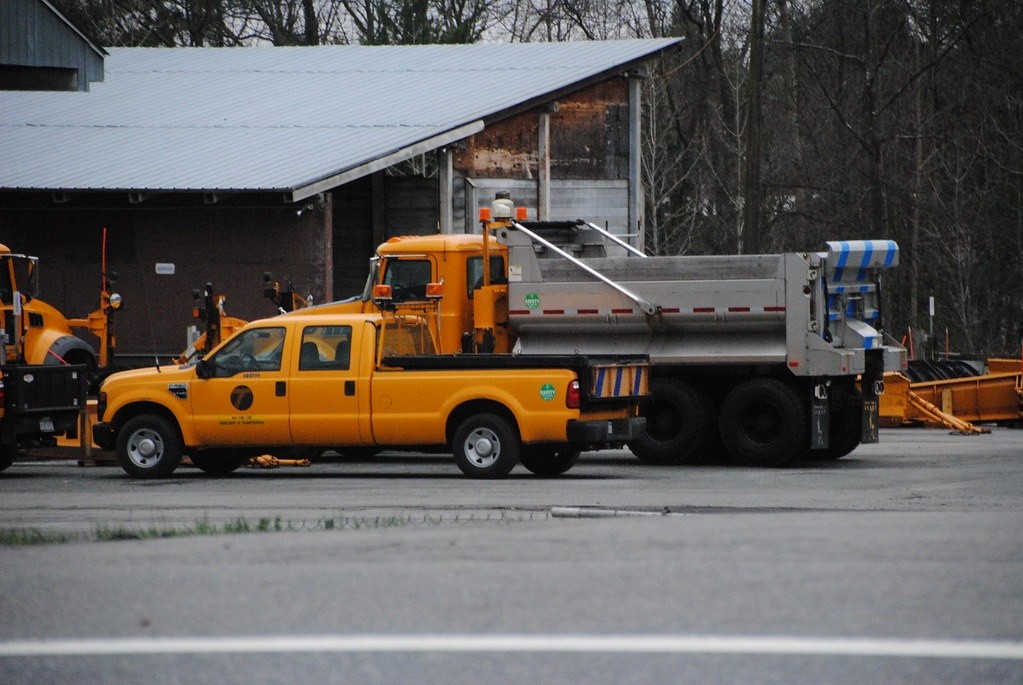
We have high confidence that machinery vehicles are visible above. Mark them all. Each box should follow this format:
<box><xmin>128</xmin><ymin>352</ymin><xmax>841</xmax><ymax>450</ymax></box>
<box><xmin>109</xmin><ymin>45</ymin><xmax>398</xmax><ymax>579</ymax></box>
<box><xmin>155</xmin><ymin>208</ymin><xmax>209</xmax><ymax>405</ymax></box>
<box><xmin>0</xmin><ymin>225</ymin><xmax>126</xmax><ymax>485</ymax></box>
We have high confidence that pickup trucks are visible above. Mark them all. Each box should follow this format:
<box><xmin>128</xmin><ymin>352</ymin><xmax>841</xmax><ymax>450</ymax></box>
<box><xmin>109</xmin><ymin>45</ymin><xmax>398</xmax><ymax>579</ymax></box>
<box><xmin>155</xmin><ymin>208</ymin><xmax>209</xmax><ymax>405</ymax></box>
<box><xmin>80</xmin><ymin>287</ymin><xmax>651</xmax><ymax>487</ymax></box>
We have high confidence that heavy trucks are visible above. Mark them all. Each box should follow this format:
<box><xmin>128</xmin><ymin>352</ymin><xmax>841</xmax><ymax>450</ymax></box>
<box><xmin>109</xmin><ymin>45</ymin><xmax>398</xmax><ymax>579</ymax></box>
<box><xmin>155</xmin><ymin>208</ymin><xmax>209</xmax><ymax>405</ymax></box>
<box><xmin>255</xmin><ymin>193</ymin><xmax>918</xmax><ymax>469</ymax></box>
